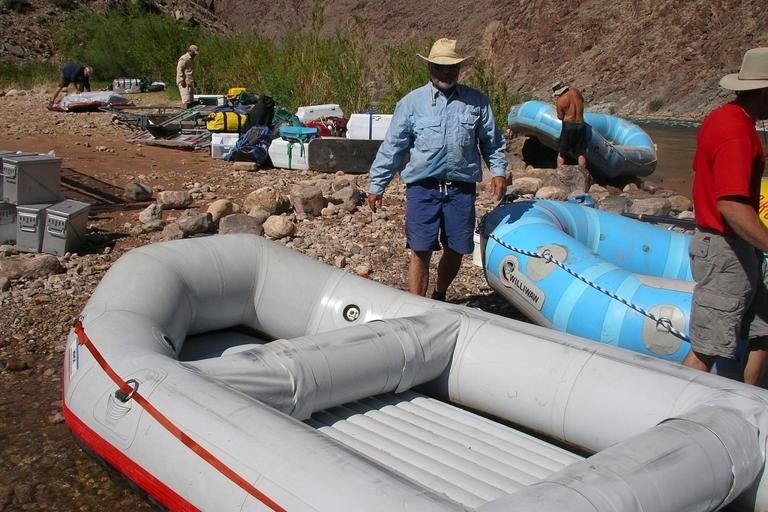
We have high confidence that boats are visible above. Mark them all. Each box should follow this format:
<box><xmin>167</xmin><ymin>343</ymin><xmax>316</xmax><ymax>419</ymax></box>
<box><xmin>509</xmin><ymin>100</ymin><xmax>658</xmax><ymax>177</ymax></box>
<box><xmin>478</xmin><ymin>200</ymin><xmax>768</xmax><ymax>387</ymax></box>
<box><xmin>63</xmin><ymin>234</ymin><xmax>768</xmax><ymax>511</ymax></box>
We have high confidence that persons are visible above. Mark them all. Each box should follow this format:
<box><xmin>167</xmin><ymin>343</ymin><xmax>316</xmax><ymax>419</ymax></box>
<box><xmin>46</xmin><ymin>63</ymin><xmax>92</xmax><ymax>110</ymax></box>
<box><xmin>551</xmin><ymin>80</ymin><xmax>587</xmax><ymax>168</ymax></box>
<box><xmin>681</xmin><ymin>48</ymin><xmax>767</xmax><ymax>385</ymax></box>
<box><xmin>175</xmin><ymin>45</ymin><xmax>199</xmax><ymax>108</ymax></box>
<box><xmin>367</xmin><ymin>39</ymin><xmax>509</xmax><ymax>300</ymax></box>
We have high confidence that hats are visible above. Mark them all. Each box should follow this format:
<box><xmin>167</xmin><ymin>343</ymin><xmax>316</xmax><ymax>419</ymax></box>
<box><xmin>719</xmin><ymin>47</ymin><xmax>768</xmax><ymax>91</ymax></box>
<box><xmin>414</xmin><ymin>37</ymin><xmax>476</xmax><ymax>67</ymax></box>
<box><xmin>189</xmin><ymin>45</ymin><xmax>200</xmax><ymax>55</ymax></box>
<box><xmin>551</xmin><ymin>80</ymin><xmax>569</xmax><ymax>97</ymax></box>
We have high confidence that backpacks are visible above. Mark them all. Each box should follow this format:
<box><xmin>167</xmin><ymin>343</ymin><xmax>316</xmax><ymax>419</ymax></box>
<box><xmin>205</xmin><ymin>88</ymin><xmax>345</xmax><ymax>170</ymax></box>
